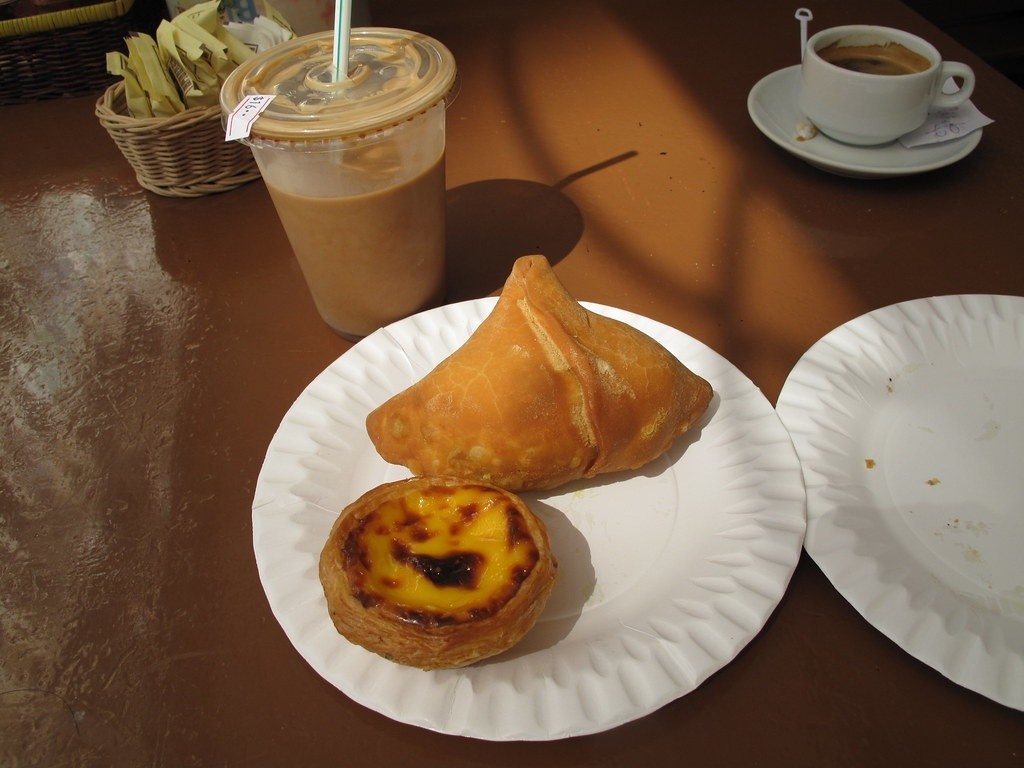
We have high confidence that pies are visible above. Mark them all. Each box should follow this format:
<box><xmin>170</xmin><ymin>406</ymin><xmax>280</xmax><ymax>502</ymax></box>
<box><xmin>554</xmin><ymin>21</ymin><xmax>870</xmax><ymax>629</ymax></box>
<box><xmin>363</xmin><ymin>254</ymin><xmax>714</xmax><ymax>491</ymax></box>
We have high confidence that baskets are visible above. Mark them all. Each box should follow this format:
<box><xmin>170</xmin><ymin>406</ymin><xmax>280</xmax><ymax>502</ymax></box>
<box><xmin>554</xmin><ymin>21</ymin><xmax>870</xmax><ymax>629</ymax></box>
<box><xmin>93</xmin><ymin>78</ymin><xmax>262</xmax><ymax>198</ymax></box>
<box><xmin>0</xmin><ymin>0</ymin><xmax>154</xmax><ymax>101</ymax></box>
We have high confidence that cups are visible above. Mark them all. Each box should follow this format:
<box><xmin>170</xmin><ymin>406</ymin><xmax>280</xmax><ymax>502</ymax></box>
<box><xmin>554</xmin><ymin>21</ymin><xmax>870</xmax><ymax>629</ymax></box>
<box><xmin>798</xmin><ymin>25</ymin><xmax>975</xmax><ymax>146</ymax></box>
<box><xmin>219</xmin><ymin>28</ymin><xmax>457</xmax><ymax>339</ymax></box>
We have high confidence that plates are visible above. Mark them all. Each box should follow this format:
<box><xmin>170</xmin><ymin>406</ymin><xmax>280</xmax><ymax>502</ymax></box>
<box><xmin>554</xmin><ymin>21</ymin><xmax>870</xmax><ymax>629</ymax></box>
<box><xmin>252</xmin><ymin>301</ymin><xmax>807</xmax><ymax>744</ymax></box>
<box><xmin>748</xmin><ymin>64</ymin><xmax>983</xmax><ymax>177</ymax></box>
<box><xmin>777</xmin><ymin>293</ymin><xmax>1024</xmax><ymax>714</ymax></box>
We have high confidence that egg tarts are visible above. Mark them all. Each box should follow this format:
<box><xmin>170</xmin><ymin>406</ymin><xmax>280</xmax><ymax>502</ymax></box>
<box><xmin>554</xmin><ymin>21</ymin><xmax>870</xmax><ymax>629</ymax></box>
<box><xmin>318</xmin><ymin>473</ymin><xmax>559</xmax><ymax>671</ymax></box>
